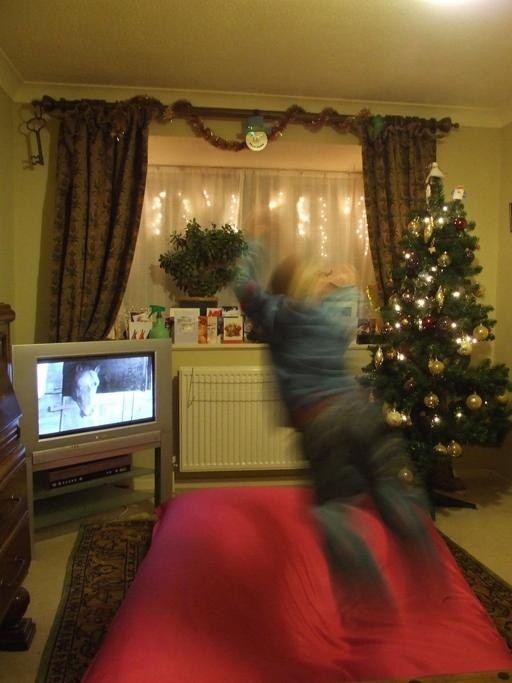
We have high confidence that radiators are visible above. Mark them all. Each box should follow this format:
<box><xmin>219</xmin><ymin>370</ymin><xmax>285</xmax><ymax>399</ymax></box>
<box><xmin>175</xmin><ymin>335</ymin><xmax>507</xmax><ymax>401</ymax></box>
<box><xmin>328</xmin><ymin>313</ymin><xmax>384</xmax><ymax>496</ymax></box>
<box><xmin>179</xmin><ymin>365</ymin><xmax>311</xmax><ymax>473</ymax></box>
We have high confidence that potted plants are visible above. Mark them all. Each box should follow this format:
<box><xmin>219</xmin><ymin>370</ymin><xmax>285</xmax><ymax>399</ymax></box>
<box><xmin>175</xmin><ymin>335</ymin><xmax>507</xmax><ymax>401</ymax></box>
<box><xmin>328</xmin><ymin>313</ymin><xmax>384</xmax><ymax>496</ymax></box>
<box><xmin>158</xmin><ymin>218</ymin><xmax>249</xmax><ymax>316</ymax></box>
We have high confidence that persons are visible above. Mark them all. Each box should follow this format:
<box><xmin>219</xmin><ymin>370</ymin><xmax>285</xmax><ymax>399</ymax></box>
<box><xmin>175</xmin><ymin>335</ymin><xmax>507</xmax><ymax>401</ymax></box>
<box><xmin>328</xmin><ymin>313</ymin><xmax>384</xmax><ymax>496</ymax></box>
<box><xmin>223</xmin><ymin>243</ymin><xmax>452</xmax><ymax>630</ymax></box>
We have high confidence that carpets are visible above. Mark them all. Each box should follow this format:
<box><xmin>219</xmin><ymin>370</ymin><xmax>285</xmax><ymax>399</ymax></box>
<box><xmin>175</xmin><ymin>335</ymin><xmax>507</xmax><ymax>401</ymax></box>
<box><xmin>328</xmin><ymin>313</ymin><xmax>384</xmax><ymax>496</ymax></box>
<box><xmin>34</xmin><ymin>514</ymin><xmax>510</xmax><ymax>683</ymax></box>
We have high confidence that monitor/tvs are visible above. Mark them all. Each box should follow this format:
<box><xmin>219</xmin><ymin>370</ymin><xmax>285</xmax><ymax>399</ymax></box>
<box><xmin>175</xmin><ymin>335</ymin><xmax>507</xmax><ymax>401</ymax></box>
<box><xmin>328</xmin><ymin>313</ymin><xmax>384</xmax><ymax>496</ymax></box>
<box><xmin>12</xmin><ymin>337</ymin><xmax>172</xmax><ymax>458</ymax></box>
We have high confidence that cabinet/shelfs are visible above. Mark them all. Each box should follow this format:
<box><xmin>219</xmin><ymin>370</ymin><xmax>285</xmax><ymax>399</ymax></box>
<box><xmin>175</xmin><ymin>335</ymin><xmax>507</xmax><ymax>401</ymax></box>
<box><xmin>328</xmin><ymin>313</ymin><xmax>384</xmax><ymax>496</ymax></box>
<box><xmin>0</xmin><ymin>302</ymin><xmax>37</xmax><ymax>653</ymax></box>
